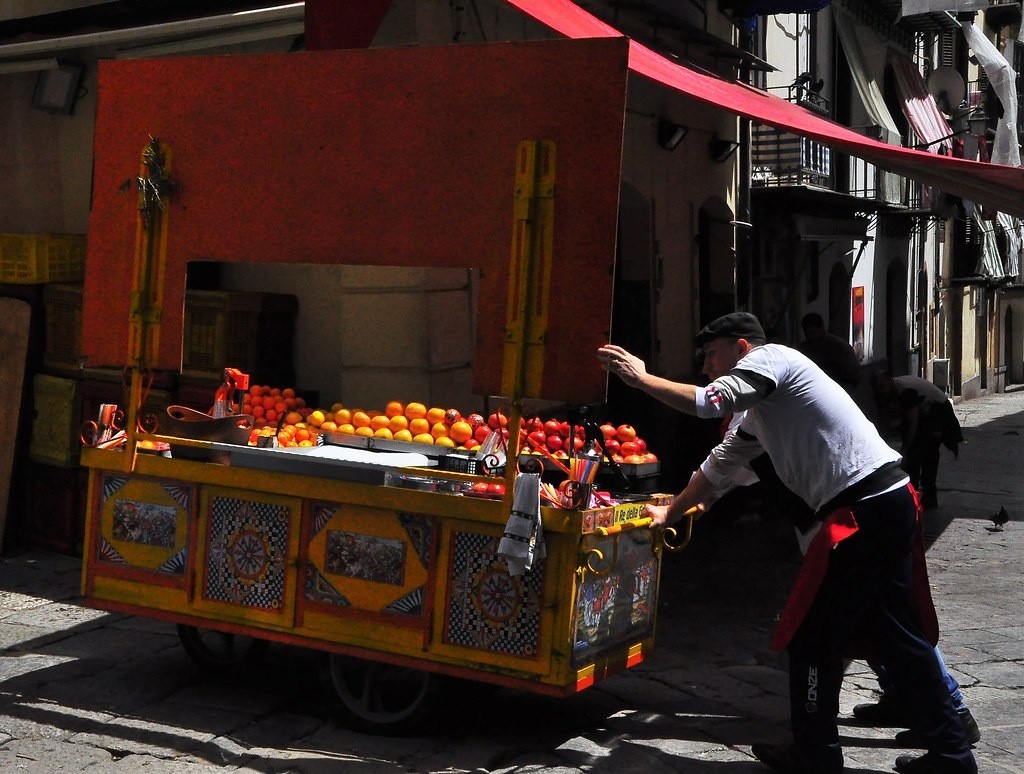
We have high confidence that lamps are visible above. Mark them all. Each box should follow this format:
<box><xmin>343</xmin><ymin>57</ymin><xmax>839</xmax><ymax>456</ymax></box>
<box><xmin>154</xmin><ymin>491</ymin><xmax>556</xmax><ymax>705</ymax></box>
<box><xmin>656</xmin><ymin>116</ymin><xmax>687</xmax><ymax>151</ymax></box>
<box><xmin>707</xmin><ymin>138</ymin><xmax>740</xmax><ymax>164</ymax></box>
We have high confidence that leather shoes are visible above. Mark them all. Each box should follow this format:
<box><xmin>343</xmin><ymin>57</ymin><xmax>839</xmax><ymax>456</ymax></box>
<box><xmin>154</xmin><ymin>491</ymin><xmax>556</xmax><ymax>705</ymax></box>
<box><xmin>751</xmin><ymin>742</ymin><xmax>844</xmax><ymax>773</ymax></box>
<box><xmin>895</xmin><ymin>752</ymin><xmax>978</xmax><ymax>774</ymax></box>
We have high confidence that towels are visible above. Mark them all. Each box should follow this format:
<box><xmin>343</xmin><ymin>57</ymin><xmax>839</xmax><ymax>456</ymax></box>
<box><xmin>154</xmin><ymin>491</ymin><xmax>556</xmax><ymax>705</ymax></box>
<box><xmin>497</xmin><ymin>472</ymin><xmax>547</xmax><ymax>577</ymax></box>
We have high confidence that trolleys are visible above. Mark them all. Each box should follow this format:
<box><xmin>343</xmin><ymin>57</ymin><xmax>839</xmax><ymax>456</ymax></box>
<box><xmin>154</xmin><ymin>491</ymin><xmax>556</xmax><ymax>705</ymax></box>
<box><xmin>81</xmin><ymin>397</ymin><xmax>708</xmax><ymax>733</ymax></box>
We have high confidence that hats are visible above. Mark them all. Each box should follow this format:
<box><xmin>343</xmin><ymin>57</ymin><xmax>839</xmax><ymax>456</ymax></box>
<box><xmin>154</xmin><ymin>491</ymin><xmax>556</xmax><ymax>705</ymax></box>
<box><xmin>694</xmin><ymin>312</ymin><xmax>766</xmax><ymax>348</ymax></box>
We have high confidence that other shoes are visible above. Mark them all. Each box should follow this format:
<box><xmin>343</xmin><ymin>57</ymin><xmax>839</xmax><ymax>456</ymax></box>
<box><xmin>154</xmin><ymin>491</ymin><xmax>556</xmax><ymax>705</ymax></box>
<box><xmin>854</xmin><ymin>689</ymin><xmax>964</xmax><ymax>724</ymax></box>
<box><xmin>896</xmin><ymin>708</ymin><xmax>980</xmax><ymax>748</ymax></box>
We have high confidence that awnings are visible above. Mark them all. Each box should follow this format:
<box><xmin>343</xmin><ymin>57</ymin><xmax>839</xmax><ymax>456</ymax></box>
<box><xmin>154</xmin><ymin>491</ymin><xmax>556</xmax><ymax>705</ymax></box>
<box><xmin>751</xmin><ymin>185</ymin><xmax>908</xmax><ymax>211</ymax></box>
<box><xmin>610</xmin><ymin>0</ymin><xmax>780</xmax><ymax>73</ymax></box>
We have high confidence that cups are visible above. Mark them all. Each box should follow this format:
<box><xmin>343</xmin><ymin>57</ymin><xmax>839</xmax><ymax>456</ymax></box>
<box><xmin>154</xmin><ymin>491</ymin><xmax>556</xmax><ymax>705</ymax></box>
<box><xmin>474</xmin><ymin>430</ymin><xmax>511</xmax><ymax>461</ymax></box>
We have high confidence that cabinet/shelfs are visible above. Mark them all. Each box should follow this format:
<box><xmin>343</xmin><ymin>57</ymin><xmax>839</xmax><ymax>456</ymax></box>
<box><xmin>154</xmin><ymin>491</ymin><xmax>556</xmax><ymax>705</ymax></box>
<box><xmin>7</xmin><ymin>359</ymin><xmax>322</xmax><ymax>565</ymax></box>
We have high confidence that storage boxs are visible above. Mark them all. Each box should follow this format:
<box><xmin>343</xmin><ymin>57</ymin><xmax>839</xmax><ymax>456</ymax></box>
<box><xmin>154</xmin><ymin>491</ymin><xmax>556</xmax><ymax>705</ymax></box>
<box><xmin>0</xmin><ymin>232</ymin><xmax>268</xmax><ymax>373</ymax></box>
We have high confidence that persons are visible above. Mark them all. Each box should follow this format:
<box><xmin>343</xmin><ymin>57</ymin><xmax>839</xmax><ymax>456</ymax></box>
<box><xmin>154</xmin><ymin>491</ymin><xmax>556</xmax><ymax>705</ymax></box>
<box><xmin>597</xmin><ymin>313</ymin><xmax>981</xmax><ymax>774</ymax></box>
<box><xmin>801</xmin><ymin>312</ymin><xmax>861</xmax><ymax>392</ymax></box>
<box><xmin>869</xmin><ymin>368</ymin><xmax>961</xmax><ymax>510</ymax></box>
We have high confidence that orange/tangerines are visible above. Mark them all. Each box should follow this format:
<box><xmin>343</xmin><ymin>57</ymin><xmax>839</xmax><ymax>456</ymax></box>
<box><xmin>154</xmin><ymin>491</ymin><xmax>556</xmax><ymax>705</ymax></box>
<box><xmin>286</xmin><ymin>398</ymin><xmax>472</xmax><ymax>449</ymax></box>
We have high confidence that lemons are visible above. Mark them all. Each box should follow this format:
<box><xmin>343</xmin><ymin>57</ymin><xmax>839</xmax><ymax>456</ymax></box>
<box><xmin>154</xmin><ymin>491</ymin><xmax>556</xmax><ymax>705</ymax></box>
<box><xmin>232</xmin><ymin>385</ymin><xmax>315</xmax><ymax>449</ymax></box>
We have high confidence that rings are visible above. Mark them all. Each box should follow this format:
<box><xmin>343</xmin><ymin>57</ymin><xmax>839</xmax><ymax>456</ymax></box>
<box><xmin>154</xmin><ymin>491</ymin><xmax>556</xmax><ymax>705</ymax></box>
<box><xmin>613</xmin><ymin>359</ymin><xmax>618</xmax><ymax>365</ymax></box>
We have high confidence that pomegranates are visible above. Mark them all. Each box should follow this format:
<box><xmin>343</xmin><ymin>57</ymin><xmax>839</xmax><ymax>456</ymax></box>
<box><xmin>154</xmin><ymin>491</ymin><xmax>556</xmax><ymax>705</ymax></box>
<box><xmin>445</xmin><ymin>409</ymin><xmax>658</xmax><ymax>495</ymax></box>
